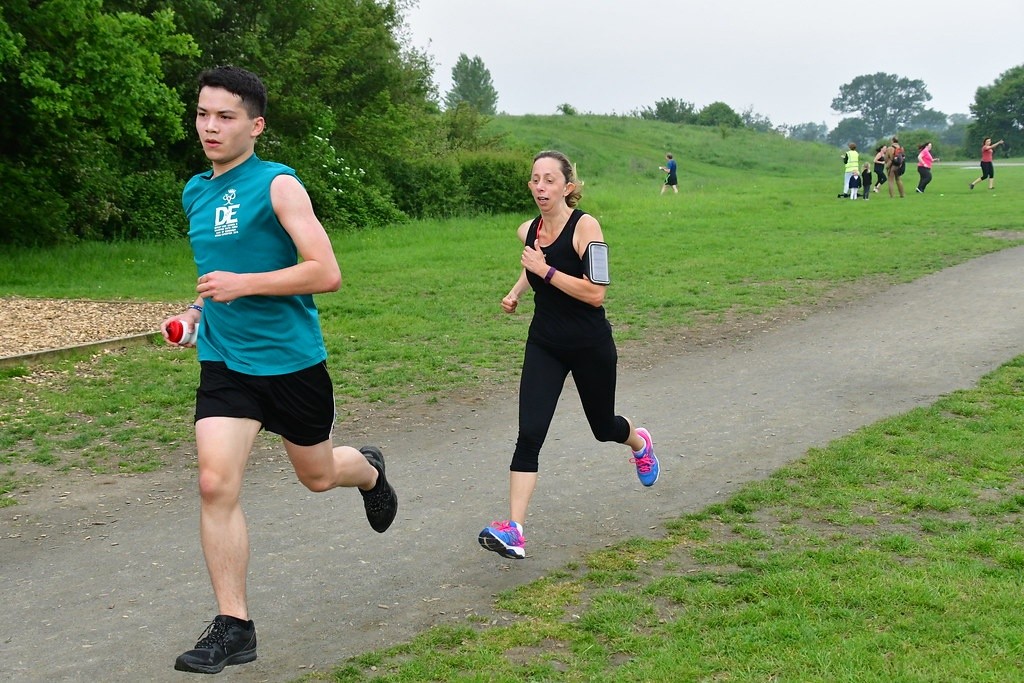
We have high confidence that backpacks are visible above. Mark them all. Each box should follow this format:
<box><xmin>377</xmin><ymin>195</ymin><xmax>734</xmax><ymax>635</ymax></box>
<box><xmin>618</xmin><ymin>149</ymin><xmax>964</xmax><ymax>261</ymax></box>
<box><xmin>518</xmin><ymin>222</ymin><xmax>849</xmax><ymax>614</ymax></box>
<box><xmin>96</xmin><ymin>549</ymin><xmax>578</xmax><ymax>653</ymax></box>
<box><xmin>889</xmin><ymin>145</ymin><xmax>905</xmax><ymax>167</ymax></box>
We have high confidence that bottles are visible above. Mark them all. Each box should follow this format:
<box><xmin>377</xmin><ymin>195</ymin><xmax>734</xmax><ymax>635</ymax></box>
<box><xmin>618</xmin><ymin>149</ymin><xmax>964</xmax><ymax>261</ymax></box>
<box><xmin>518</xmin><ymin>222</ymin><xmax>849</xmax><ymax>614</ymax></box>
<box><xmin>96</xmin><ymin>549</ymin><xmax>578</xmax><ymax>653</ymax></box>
<box><xmin>166</xmin><ymin>319</ymin><xmax>199</xmax><ymax>346</ymax></box>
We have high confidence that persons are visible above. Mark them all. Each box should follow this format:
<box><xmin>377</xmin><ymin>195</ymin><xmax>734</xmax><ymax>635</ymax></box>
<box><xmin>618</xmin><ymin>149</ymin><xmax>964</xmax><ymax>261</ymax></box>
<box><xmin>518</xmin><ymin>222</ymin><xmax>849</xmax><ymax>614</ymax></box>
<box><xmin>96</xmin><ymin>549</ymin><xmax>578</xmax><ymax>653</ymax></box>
<box><xmin>862</xmin><ymin>162</ymin><xmax>872</xmax><ymax>199</ymax></box>
<box><xmin>969</xmin><ymin>137</ymin><xmax>1004</xmax><ymax>189</ymax></box>
<box><xmin>916</xmin><ymin>142</ymin><xmax>940</xmax><ymax>193</ymax></box>
<box><xmin>849</xmin><ymin>169</ymin><xmax>862</xmax><ymax>200</ymax></box>
<box><xmin>161</xmin><ymin>66</ymin><xmax>398</xmax><ymax>675</ymax></box>
<box><xmin>841</xmin><ymin>142</ymin><xmax>859</xmax><ymax>194</ymax></box>
<box><xmin>885</xmin><ymin>136</ymin><xmax>904</xmax><ymax>198</ymax></box>
<box><xmin>873</xmin><ymin>144</ymin><xmax>887</xmax><ymax>192</ymax></box>
<box><xmin>661</xmin><ymin>154</ymin><xmax>678</xmax><ymax>193</ymax></box>
<box><xmin>478</xmin><ymin>150</ymin><xmax>660</xmax><ymax>559</ymax></box>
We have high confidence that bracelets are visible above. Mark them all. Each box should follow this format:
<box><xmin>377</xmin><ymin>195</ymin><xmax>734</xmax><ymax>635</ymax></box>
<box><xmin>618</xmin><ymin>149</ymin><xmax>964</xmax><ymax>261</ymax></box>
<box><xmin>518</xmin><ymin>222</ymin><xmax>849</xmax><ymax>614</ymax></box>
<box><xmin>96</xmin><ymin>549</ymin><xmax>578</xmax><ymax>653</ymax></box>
<box><xmin>190</xmin><ymin>305</ymin><xmax>202</xmax><ymax>312</ymax></box>
<box><xmin>545</xmin><ymin>267</ymin><xmax>556</xmax><ymax>282</ymax></box>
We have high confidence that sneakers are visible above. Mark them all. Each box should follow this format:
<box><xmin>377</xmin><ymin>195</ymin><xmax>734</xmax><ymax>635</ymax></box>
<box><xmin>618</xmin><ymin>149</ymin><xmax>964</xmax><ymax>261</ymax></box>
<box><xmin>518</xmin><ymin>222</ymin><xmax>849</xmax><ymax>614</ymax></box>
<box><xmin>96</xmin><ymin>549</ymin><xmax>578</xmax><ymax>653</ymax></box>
<box><xmin>479</xmin><ymin>520</ymin><xmax>525</xmax><ymax>559</ymax></box>
<box><xmin>174</xmin><ymin>616</ymin><xmax>257</xmax><ymax>674</ymax></box>
<box><xmin>358</xmin><ymin>446</ymin><xmax>397</xmax><ymax>533</ymax></box>
<box><xmin>629</xmin><ymin>428</ymin><xmax>660</xmax><ymax>487</ymax></box>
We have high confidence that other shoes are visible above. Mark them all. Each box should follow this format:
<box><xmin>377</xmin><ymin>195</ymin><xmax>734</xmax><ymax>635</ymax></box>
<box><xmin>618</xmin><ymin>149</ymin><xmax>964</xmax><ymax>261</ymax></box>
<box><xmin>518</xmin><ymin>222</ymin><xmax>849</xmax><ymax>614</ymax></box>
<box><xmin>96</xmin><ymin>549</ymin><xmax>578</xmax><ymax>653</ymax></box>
<box><xmin>916</xmin><ymin>188</ymin><xmax>923</xmax><ymax>192</ymax></box>
<box><xmin>874</xmin><ymin>187</ymin><xmax>879</xmax><ymax>192</ymax></box>
<box><xmin>970</xmin><ymin>184</ymin><xmax>974</xmax><ymax>189</ymax></box>
